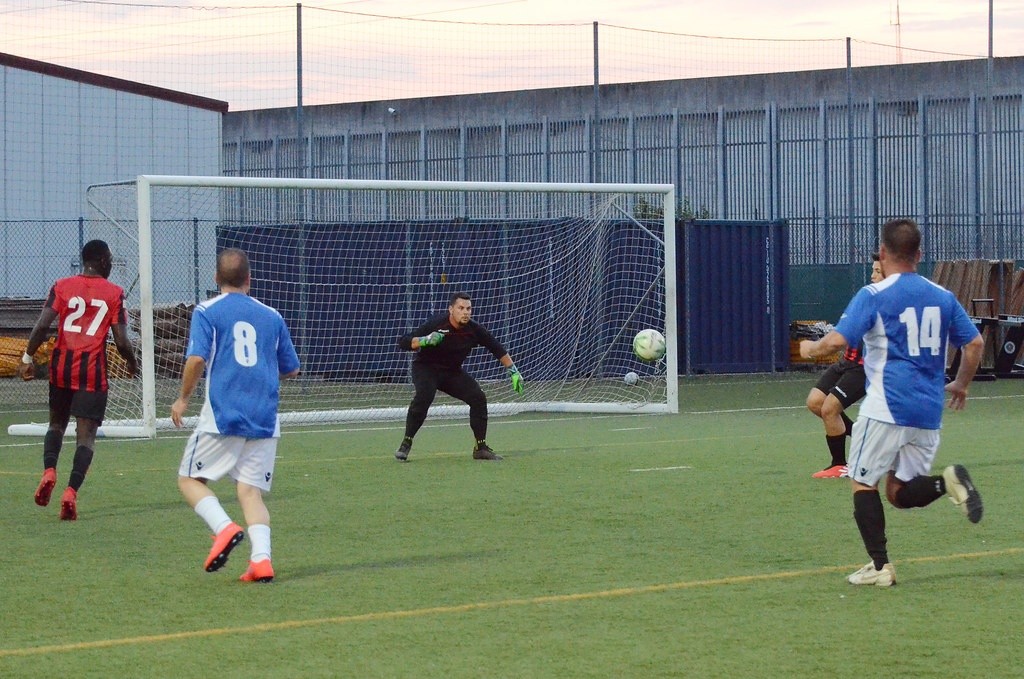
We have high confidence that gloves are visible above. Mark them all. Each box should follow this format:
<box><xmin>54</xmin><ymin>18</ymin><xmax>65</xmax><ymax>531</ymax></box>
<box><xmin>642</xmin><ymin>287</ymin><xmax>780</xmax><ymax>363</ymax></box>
<box><xmin>419</xmin><ymin>332</ymin><xmax>444</xmax><ymax>345</ymax></box>
<box><xmin>508</xmin><ymin>363</ymin><xmax>524</xmax><ymax>396</ymax></box>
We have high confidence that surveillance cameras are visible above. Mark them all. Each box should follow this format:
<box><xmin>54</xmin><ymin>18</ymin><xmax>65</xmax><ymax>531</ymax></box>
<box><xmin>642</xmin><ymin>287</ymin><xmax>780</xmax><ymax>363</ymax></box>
<box><xmin>388</xmin><ymin>108</ymin><xmax>396</xmax><ymax>114</ymax></box>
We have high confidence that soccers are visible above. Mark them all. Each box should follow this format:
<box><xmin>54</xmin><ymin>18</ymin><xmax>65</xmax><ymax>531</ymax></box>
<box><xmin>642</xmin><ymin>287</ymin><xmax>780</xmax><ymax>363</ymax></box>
<box><xmin>632</xmin><ymin>329</ymin><xmax>666</xmax><ymax>362</ymax></box>
<box><xmin>624</xmin><ymin>371</ymin><xmax>639</xmax><ymax>386</ymax></box>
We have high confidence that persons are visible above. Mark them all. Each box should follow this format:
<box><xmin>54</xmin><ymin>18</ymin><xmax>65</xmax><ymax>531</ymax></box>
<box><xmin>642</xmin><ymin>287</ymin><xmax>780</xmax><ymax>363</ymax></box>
<box><xmin>170</xmin><ymin>247</ymin><xmax>301</xmax><ymax>581</ymax></box>
<box><xmin>395</xmin><ymin>293</ymin><xmax>524</xmax><ymax>459</ymax></box>
<box><xmin>803</xmin><ymin>251</ymin><xmax>884</xmax><ymax>480</ymax></box>
<box><xmin>798</xmin><ymin>219</ymin><xmax>984</xmax><ymax>590</ymax></box>
<box><xmin>20</xmin><ymin>240</ymin><xmax>137</xmax><ymax>520</ymax></box>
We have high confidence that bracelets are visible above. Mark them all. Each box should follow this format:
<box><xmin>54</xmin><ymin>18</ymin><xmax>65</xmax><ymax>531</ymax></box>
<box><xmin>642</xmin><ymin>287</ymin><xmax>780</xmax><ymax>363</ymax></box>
<box><xmin>807</xmin><ymin>355</ymin><xmax>817</xmax><ymax>361</ymax></box>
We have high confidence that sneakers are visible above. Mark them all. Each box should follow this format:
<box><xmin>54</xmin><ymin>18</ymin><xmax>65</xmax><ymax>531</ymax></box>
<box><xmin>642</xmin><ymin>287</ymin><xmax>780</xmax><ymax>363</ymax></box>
<box><xmin>473</xmin><ymin>442</ymin><xmax>503</xmax><ymax>460</ymax></box>
<box><xmin>812</xmin><ymin>462</ymin><xmax>848</xmax><ymax>480</ymax></box>
<box><xmin>61</xmin><ymin>487</ymin><xmax>78</xmax><ymax>521</ymax></box>
<box><xmin>239</xmin><ymin>556</ymin><xmax>275</xmax><ymax>583</ymax></box>
<box><xmin>34</xmin><ymin>468</ymin><xmax>57</xmax><ymax>506</ymax></box>
<box><xmin>848</xmin><ymin>560</ymin><xmax>897</xmax><ymax>586</ymax></box>
<box><xmin>395</xmin><ymin>437</ymin><xmax>412</xmax><ymax>460</ymax></box>
<box><xmin>204</xmin><ymin>522</ymin><xmax>245</xmax><ymax>573</ymax></box>
<box><xmin>942</xmin><ymin>464</ymin><xmax>982</xmax><ymax>523</ymax></box>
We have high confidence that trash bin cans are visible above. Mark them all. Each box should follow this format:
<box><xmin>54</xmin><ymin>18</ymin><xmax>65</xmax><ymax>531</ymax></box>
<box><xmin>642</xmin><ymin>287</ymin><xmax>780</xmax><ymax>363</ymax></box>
<box><xmin>788</xmin><ymin>318</ymin><xmax>839</xmax><ymax>371</ymax></box>
